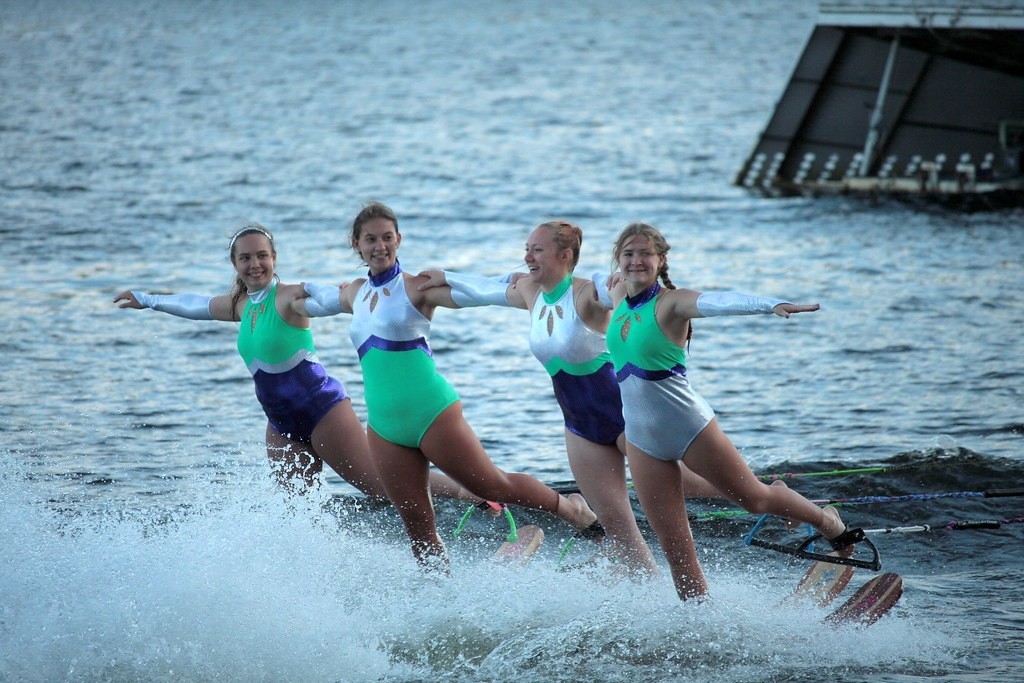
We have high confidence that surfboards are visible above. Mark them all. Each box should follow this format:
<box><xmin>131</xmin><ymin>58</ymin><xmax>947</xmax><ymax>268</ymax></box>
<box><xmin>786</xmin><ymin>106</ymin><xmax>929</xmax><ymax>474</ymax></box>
<box><xmin>490</xmin><ymin>523</ymin><xmax>904</xmax><ymax>628</ymax></box>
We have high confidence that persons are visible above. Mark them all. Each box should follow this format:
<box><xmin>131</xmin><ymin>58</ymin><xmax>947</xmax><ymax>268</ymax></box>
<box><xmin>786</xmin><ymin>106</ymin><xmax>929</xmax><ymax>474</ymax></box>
<box><xmin>113</xmin><ymin>224</ymin><xmax>501</xmax><ymax>517</ymax></box>
<box><xmin>591</xmin><ymin>223</ymin><xmax>856</xmax><ymax>605</ymax></box>
<box><xmin>294</xmin><ymin>202</ymin><xmax>607</xmax><ymax>576</ymax></box>
<box><xmin>417</xmin><ymin>223</ymin><xmax>800</xmax><ymax>586</ymax></box>
